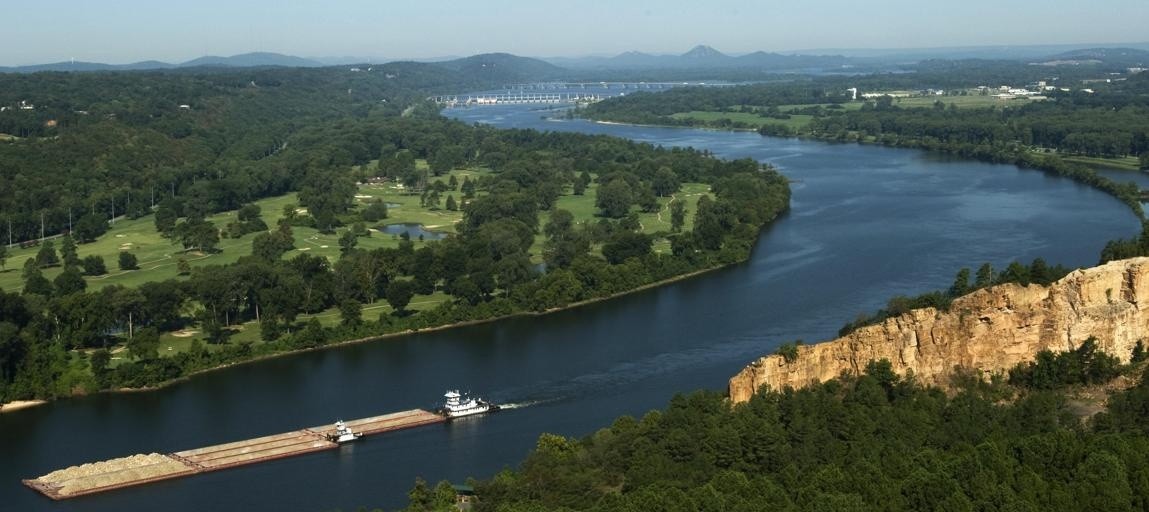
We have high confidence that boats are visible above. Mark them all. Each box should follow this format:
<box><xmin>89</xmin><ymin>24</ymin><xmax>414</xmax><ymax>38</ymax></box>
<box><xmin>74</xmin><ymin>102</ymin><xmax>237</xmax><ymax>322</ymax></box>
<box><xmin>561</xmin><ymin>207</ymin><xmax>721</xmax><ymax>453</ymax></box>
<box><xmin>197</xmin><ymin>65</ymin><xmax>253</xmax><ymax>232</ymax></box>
<box><xmin>326</xmin><ymin>419</ymin><xmax>367</xmax><ymax>444</ymax></box>
<box><xmin>437</xmin><ymin>388</ymin><xmax>498</xmax><ymax>422</ymax></box>
